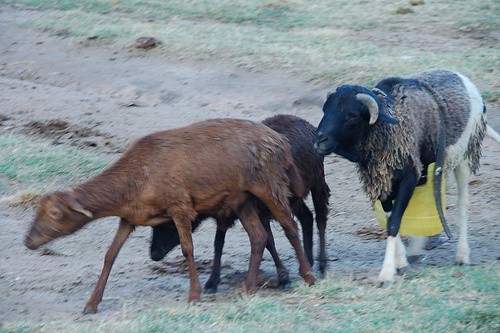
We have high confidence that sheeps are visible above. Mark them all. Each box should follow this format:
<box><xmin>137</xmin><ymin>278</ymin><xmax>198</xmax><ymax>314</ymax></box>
<box><xmin>150</xmin><ymin>114</ymin><xmax>331</xmax><ymax>293</ymax></box>
<box><xmin>315</xmin><ymin>68</ymin><xmax>500</xmax><ymax>289</ymax></box>
<box><xmin>24</xmin><ymin>118</ymin><xmax>316</xmax><ymax>314</ymax></box>
